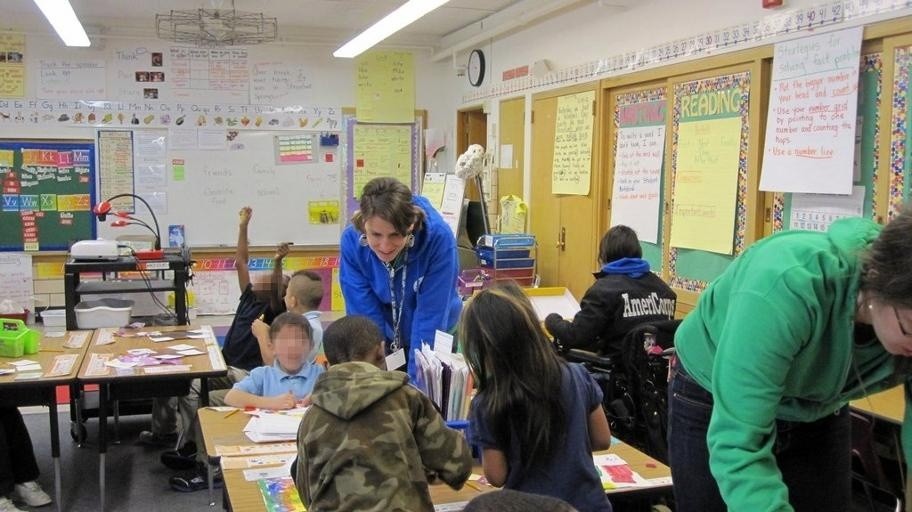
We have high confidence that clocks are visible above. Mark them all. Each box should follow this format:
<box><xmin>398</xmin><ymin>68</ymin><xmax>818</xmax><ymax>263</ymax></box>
<box><xmin>467</xmin><ymin>48</ymin><xmax>485</xmax><ymax>87</ymax></box>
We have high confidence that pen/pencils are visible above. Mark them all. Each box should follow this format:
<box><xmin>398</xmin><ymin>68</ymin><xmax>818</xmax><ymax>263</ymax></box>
<box><xmin>224</xmin><ymin>408</ymin><xmax>240</xmax><ymax>419</ymax></box>
<box><xmin>465</xmin><ymin>481</ymin><xmax>482</xmax><ymax>493</ymax></box>
<box><xmin>39</xmin><ymin>349</ymin><xmax>65</xmax><ymax>352</ymax></box>
<box><xmin>289</xmin><ymin>390</ymin><xmax>293</xmax><ymax>394</ymax></box>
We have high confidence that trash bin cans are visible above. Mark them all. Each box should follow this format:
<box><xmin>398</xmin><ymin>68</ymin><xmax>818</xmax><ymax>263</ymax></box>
<box><xmin>154</xmin><ymin>313</ymin><xmax>177</xmax><ymax>325</ymax></box>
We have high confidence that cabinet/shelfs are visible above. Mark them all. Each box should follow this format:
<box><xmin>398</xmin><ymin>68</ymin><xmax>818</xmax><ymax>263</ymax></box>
<box><xmin>848</xmin><ymin>383</ymin><xmax>905</xmax><ymax>512</ymax></box>
<box><xmin>476</xmin><ymin>234</ymin><xmax>537</xmax><ymax>288</ymax></box>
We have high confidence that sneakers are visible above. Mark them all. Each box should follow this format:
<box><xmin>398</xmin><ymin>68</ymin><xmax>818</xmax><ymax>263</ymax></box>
<box><xmin>161</xmin><ymin>440</ymin><xmax>199</xmax><ymax>467</ymax></box>
<box><xmin>141</xmin><ymin>429</ymin><xmax>176</xmax><ymax>446</ymax></box>
<box><xmin>169</xmin><ymin>468</ymin><xmax>223</xmax><ymax>491</ymax></box>
<box><xmin>0</xmin><ymin>479</ymin><xmax>53</xmax><ymax>511</ymax></box>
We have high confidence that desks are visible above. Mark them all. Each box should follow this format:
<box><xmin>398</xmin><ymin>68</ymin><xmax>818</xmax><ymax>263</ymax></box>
<box><xmin>218</xmin><ymin>430</ymin><xmax>677</xmax><ymax>512</ymax></box>
<box><xmin>76</xmin><ymin>325</ymin><xmax>228</xmax><ymax>512</ymax></box>
<box><xmin>0</xmin><ymin>330</ymin><xmax>94</xmax><ymax>512</ymax></box>
<box><xmin>197</xmin><ymin>405</ymin><xmax>313</xmax><ymax>509</ymax></box>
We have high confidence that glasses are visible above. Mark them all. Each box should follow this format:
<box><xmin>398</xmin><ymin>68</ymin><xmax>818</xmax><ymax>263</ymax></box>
<box><xmin>890</xmin><ymin>297</ymin><xmax>911</xmax><ymax>336</ymax></box>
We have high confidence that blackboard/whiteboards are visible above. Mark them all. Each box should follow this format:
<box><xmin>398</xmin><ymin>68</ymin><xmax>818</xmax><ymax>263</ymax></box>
<box><xmin>0</xmin><ymin>127</ymin><xmax>347</xmax><ymax>253</ymax></box>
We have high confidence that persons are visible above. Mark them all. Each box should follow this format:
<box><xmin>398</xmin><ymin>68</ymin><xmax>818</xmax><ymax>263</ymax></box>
<box><xmin>1</xmin><ymin>402</ymin><xmax>54</xmax><ymax>511</ymax></box>
<box><xmin>157</xmin><ymin>270</ymin><xmax>325</xmax><ymax>493</ymax></box>
<box><xmin>545</xmin><ymin>224</ymin><xmax>677</xmax><ymax>369</ymax></box>
<box><xmin>666</xmin><ymin>197</ymin><xmax>911</xmax><ymax>512</ymax></box>
<box><xmin>222</xmin><ymin>314</ymin><xmax>329</xmax><ymax>410</ymax></box>
<box><xmin>457</xmin><ymin>278</ymin><xmax>616</xmax><ymax>511</ymax></box>
<box><xmin>293</xmin><ymin>314</ymin><xmax>475</xmax><ymax>511</ymax></box>
<box><xmin>137</xmin><ymin>205</ymin><xmax>293</xmax><ymax>446</ymax></box>
<box><xmin>337</xmin><ymin>175</ymin><xmax>467</xmax><ymax>388</ymax></box>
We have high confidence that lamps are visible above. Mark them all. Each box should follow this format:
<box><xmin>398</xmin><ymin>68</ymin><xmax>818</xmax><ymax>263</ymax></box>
<box><xmin>333</xmin><ymin>0</ymin><xmax>449</xmax><ymax>58</ymax></box>
